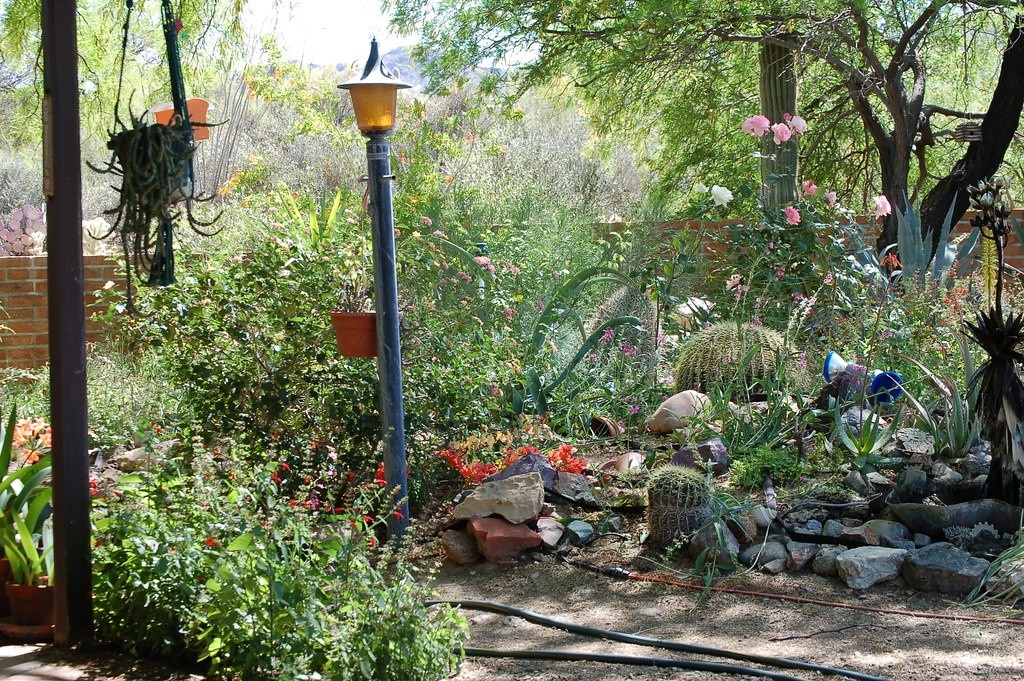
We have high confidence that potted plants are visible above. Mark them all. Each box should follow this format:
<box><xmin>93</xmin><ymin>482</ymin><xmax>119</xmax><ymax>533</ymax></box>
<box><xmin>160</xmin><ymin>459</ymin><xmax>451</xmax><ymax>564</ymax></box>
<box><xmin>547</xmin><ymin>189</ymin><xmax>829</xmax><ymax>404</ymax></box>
<box><xmin>0</xmin><ymin>402</ymin><xmax>61</xmax><ymax>639</ymax></box>
<box><xmin>330</xmin><ymin>259</ymin><xmax>379</xmax><ymax>359</ymax></box>
<box><xmin>86</xmin><ymin>89</ymin><xmax>229</xmax><ymax>320</ymax></box>
